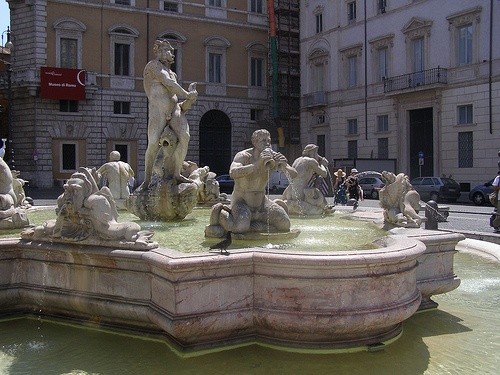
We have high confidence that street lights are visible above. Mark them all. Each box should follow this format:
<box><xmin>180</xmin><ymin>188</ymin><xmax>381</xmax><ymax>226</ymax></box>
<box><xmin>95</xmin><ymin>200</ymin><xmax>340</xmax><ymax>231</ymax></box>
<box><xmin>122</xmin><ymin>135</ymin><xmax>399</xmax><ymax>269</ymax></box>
<box><xmin>2</xmin><ymin>26</ymin><xmax>17</xmax><ymax>172</ymax></box>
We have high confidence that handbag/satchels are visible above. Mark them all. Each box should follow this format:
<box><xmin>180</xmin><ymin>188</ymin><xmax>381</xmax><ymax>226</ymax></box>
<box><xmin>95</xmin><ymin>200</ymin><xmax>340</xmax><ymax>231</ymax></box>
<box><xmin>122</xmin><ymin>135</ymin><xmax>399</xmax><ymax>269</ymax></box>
<box><xmin>358</xmin><ymin>187</ymin><xmax>364</xmax><ymax>201</ymax></box>
<box><xmin>489</xmin><ymin>191</ymin><xmax>498</xmax><ymax>206</ymax></box>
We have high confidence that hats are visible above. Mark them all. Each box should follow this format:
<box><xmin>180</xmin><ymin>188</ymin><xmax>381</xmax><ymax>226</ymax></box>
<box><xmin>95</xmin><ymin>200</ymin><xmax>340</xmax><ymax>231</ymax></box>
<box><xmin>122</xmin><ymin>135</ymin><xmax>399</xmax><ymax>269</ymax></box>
<box><xmin>334</xmin><ymin>169</ymin><xmax>346</xmax><ymax>177</ymax></box>
<box><xmin>350</xmin><ymin>168</ymin><xmax>358</xmax><ymax>174</ymax></box>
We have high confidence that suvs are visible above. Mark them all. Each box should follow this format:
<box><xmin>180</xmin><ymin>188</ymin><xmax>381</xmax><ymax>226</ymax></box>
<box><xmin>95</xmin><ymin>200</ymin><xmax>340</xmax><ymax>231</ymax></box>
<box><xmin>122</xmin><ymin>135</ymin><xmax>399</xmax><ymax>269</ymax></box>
<box><xmin>410</xmin><ymin>177</ymin><xmax>461</xmax><ymax>204</ymax></box>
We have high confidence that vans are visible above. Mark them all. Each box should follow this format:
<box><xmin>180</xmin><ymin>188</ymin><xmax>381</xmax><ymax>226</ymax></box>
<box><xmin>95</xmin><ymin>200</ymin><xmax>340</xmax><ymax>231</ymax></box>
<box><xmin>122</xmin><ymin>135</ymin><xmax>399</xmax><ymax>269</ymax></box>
<box><xmin>266</xmin><ymin>170</ymin><xmax>291</xmax><ymax>195</ymax></box>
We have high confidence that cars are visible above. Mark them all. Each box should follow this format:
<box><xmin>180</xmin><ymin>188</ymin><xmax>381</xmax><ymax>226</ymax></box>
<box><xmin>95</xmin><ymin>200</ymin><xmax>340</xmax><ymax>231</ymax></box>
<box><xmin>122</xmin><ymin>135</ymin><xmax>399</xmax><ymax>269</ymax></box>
<box><xmin>215</xmin><ymin>175</ymin><xmax>235</xmax><ymax>194</ymax></box>
<box><xmin>469</xmin><ymin>176</ymin><xmax>496</xmax><ymax>206</ymax></box>
<box><xmin>359</xmin><ymin>177</ymin><xmax>387</xmax><ymax>198</ymax></box>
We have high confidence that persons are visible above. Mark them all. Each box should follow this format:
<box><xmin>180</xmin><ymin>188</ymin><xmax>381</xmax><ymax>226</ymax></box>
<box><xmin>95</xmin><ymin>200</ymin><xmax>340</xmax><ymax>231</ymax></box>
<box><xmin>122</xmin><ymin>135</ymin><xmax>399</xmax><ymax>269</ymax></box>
<box><xmin>212</xmin><ymin>130</ymin><xmax>298</xmax><ymax>234</ymax></box>
<box><xmin>346</xmin><ymin>168</ymin><xmax>360</xmax><ymax>201</ymax></box>
<box><xmin>282</xmin><ymin>144</ymin><xmax>328</xmax><ymax>201</ymax></box>
<box><xmin>134</xmin><ymin>40</ymin><xmax>199</xmax><ymax>195</ymax></box>
<box><xmin>97</xmin><ymin>151</ymin><xmax>134</xmax><ymax>198</ymax></box>
<box><xmin>334</xmin><ymin>169</ymin><xmax>346</xmax><ymax>206</ymax></box>
<box><xmin>380</xmin><ymin>171</ymin><xmax>428</xmax><ymax>227</ymax></box>
<box><xmin>491</xmin><ymin>175</ymin><xmax>500</xmax><ymax>232</ymax></box>
